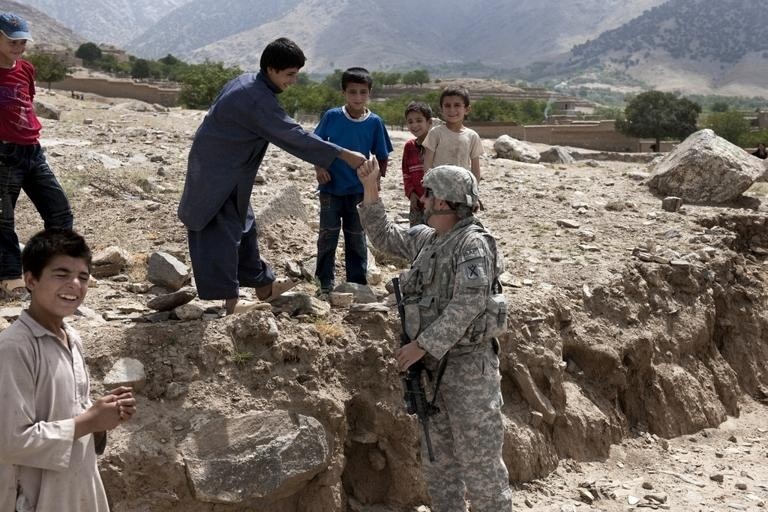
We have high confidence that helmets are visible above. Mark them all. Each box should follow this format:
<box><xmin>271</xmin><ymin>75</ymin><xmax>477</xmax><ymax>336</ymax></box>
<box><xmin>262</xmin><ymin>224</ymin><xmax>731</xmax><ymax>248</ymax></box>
<box><xmin>423</xmin><ymin>165</ymin><xmax>479</xmax><ymax>206</ymax></box>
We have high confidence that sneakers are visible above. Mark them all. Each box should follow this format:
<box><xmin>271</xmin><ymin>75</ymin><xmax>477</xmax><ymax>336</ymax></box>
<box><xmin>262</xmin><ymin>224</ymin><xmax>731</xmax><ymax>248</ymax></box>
<box><xmin>316</xmin><ymin>280</ymin><xmax>334</xmax><ymax>297</ymax></box>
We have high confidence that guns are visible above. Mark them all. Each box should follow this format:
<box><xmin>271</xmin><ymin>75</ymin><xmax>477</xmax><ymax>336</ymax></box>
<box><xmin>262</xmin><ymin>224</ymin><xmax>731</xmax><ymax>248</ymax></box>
<box><xmin>392</xmin><ymin>278</ymin><xmax>440</xmax><ymax>463</ymax></box>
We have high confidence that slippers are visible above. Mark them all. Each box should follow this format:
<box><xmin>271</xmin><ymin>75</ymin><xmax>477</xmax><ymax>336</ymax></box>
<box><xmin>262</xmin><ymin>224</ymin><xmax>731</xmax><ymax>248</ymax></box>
<box><xmin>265</xmin><ymin>276</ymin><xmax>302</xmax><ymax>303</ymax></box>
<box><xmin>1</xmin><ymin>279</ymin><xmax>26</xmax><ymax>296</ymax></box>
<box><xmin>234</xmin><ymin>299</ymin><xmax>272</xmax><ymax>313</ymax></box>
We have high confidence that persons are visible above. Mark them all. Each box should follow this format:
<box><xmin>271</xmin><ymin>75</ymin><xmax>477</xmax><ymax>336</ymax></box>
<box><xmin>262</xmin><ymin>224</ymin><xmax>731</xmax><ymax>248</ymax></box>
<box><xmin>420</xmin><ymin>85</ymin><xmax>485</xmax><ymax>183</ymax></box>
<box><xmin>313</xmin><ymin>68</ymin><xmax>393</xmax><ymax>298</ymax></box>
<box><xmin>179</xmin><ymin>37</ymin><xmax>367</xmax><ymax>315</ymax></box>
<box><xmin>750</xmin><ymin>143</ymin><xmax>768</xmax><ymax>159</ymax></box>
<box><xmin>0</xmin><ymin>12</ymin><xmax>73</xmax><ymax>298</ymax></box>
<box><xmin>355</xmin><ymin>154</ymin><xmax>513</xmax><ymax>512</ymax></box>
<box><xmin>0</xmin><ymin>227</ymin><xmax>137</xmax><ymax>512</ymax></box>
<box><xmin>402</xmin><ymin>100</ymin><xmax>433</xmax><ymax>228</ymax></box>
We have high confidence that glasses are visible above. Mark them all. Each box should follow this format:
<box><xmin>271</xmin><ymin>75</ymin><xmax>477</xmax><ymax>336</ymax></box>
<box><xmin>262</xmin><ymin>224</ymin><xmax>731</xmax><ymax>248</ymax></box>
<box><xmin>425</xmin><ymin>190</ymin><xmax>433</xmax><ymax>198</ymax></box>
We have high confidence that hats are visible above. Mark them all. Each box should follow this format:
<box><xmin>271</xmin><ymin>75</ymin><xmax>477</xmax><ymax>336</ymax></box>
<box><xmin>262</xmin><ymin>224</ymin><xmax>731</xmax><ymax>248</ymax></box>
<box><xmin>0</xmin><ymin>13</ymin><xmax>33</xmax><ymax>43</ymax></box>
